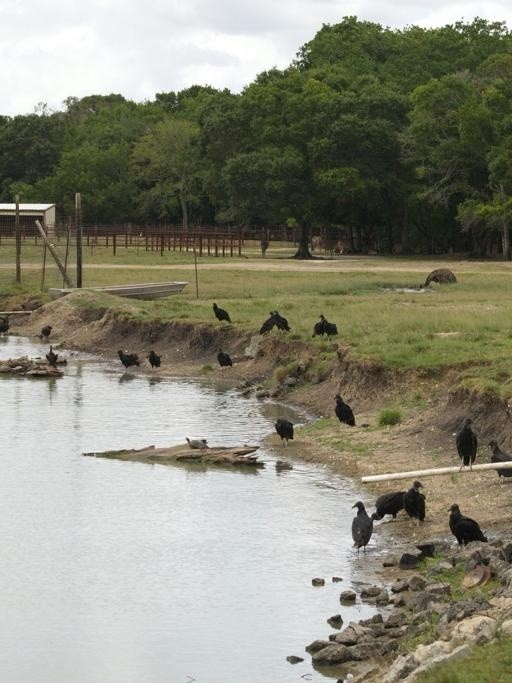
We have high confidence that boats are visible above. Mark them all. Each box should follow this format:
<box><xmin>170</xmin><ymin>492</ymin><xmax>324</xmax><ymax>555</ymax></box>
<box><xmin>48</xmin><ymin>281</ymin><xmax>188</xmax><ymax>301</ymax></box>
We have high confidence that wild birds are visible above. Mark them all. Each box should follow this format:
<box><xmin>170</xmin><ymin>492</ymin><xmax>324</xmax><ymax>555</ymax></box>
<box><xmin>259</xmin><ymin>309</ymin><xmax>291</xmax><ymax>336</ymax></box>
<box><xmin>313</xmin><ymin>315</ymin><xmax>338</xmax><ymax>337</ymax></box>
<box><xmin>212</xmin><ymin>301</ymin><xmax>232</xmax><ymax>324</ymax></box>
<box><xmin>216</xmin><ymin>347</ymin><xmax>234</xmax><ymax>368</ymax></box>
<box><xmin>117</xmin><ymin>350</ymin><xmax>161</xmax><ymax>369</ymax></box>
<box><xmin>37</xmin><ymin>325</ymin><xmax>58</xmax><ymax>369</ymax></box>
<box><xmin>186</xmin><ymin>437</ymin><xmax>209</xmax><ymax>451</ymax></box>
<box><xmin>455</xmin><ymin>418</ymin><xmax>512</xmax><ymax>485</ymax></box>
<box><xmin>334</xmin><ymin>394</ymin><xmax>355</xmax><ymax>427</ymax></box>
<box><xmin>0</xmin><ymin>316</ymin><xmax>9</xmax><ymax>334</ymax></box>
<box><xmin>351</xmin><ymin>480</ymin><xmax>489</xmax><ymax>553</ymax></box>
<box><xmin>274</xmin><ymin>419</ymin><xmax>294</xmax><ymax>447</ymax></box>
